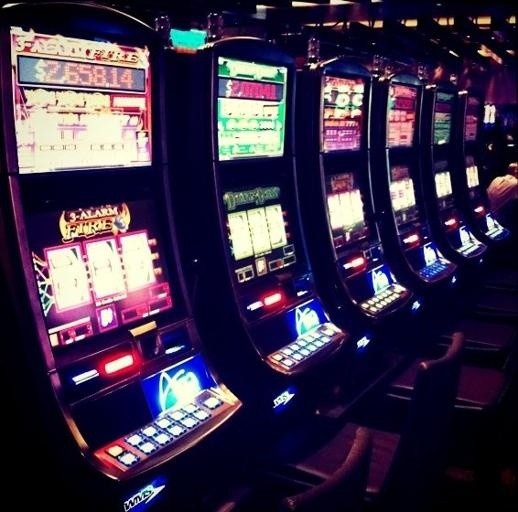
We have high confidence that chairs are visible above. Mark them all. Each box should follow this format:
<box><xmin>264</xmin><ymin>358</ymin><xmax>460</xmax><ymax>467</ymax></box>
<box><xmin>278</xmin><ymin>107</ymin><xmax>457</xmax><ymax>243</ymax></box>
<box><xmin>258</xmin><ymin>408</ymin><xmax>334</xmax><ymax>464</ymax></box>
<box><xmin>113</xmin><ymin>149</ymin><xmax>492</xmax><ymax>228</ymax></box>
<box><xmin>278</xmin><ymin>426</ymin><xmax>374</xmax><ymax>512</ymax></box>
<box><xmin>294</xmin><ymin>331</ymin><xmax>466</xmax><ymax>503</ymax></box>
<box><xmin>386</xmin><ymin>261</ymin><xmax>518</xmax><ymax>417</ymax></box>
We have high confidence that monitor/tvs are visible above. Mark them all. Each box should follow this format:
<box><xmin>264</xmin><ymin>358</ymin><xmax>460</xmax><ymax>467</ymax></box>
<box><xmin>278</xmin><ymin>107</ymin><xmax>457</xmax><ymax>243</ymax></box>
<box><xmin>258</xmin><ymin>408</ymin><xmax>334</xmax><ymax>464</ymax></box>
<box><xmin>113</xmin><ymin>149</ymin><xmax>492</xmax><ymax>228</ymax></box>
<box><xmin>217</xmin><ymin>55</ymin><xmax>289</xmax><ymax>163</ymax></box>
<box><xmin>465</xmin><ymin>163</ymin><xmax>481</xmax><ymax>192</ymax></box>
<box><xmin>434</xmin><ymin>170</ymin><xmax>453</xmax><ymax>205</ymax></box>
<box><xmin>386</xmin><ymin>84</ymin><xmax>420</xmax><ymax>148</ymax></box>
<box><xmin>434</xmin><ymin>101</ymin><xmax>453</xmax><ymax>147</ymax></box>
<box><xmin>26</xmin><ymin>204</ymin><xmax>175</xmax><ymax>351</ymax></box>
<box><xmin>7</xmin><ymin>23</ymin><xmax>155</xmax><ymax>177</ymax></box>
<box><xmin>321</xmin><ymin>73</ymin><xmax>366</xmax><ymax>156</ymax></box>
<box><xmin>221</xmin><ymin>184</ymin><xmax>300</xmax><ymax>292</ymax></box>
<box><xmin>389</xmin><ymin>175</ymin><xmax>420</xmax><ymax>223</ymax></box>
<box><xmin>327</xmin><ymin>187</ymin><xmax>374</xmax><ymax>253</ymax></box>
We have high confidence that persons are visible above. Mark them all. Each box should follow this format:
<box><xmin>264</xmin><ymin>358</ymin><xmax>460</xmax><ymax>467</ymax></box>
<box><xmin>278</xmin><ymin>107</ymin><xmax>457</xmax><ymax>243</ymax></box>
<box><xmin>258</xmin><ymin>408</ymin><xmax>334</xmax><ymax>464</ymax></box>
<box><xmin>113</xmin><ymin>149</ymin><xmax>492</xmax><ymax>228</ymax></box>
<box><xmin>487</xmin><ymin>162</ymin><xmax>518</xmax><ymax>213</ymax></box>
